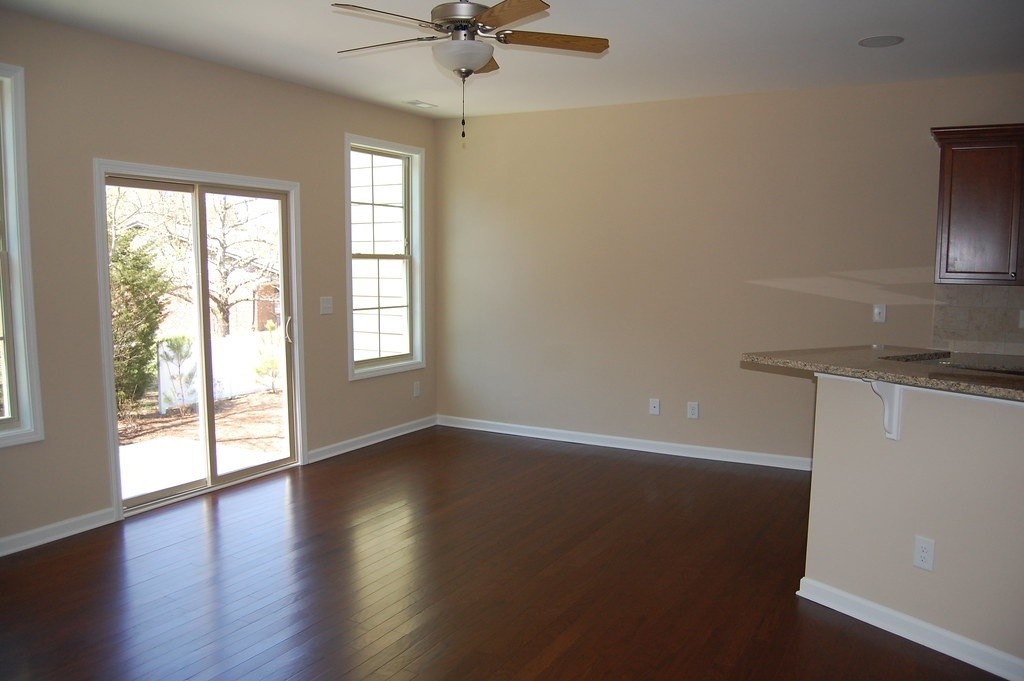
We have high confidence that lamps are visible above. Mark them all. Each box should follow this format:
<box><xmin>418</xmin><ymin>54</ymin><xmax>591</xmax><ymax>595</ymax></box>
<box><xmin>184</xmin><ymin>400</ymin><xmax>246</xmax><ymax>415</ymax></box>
<box><xmin>430</xmin><ymin>39</ymin><xmax>493</xmax><ymax>137</ymax></box>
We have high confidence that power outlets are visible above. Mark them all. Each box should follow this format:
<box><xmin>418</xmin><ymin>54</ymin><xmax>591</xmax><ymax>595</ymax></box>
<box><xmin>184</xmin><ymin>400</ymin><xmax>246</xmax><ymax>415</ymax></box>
<box><xmin>913</xmin><ymin>535</ymin><xmax>935</xmax><ymax>573</ymax></box>
<box><xmin>872</xmin><ymin>302</ymin><xmax>886</xmax><ymax>322</ymax></box>
<box><xmin>649</xmin><ymin>397</ymin><xmax>660</xmax><ymax>415</ymax></box>
<box><xmin>687</xmin><ymin>401</ymin><xmax>698</xmax><ymax>418</ymax></box>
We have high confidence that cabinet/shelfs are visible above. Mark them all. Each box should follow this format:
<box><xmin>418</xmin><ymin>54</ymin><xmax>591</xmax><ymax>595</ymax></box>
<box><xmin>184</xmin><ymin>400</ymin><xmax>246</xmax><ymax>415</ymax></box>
<box><xmin>930</xmin><ymin>121</ymin><xmax>1024</xmax><ymax>284</ymax></box>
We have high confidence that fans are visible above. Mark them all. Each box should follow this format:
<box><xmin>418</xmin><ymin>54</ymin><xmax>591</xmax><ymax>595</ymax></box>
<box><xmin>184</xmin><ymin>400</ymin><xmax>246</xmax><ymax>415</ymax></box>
<box><xmin>331</xmin><ymin>0</ymin><xmax>610</xmax><ymax>74</ymax></box>
